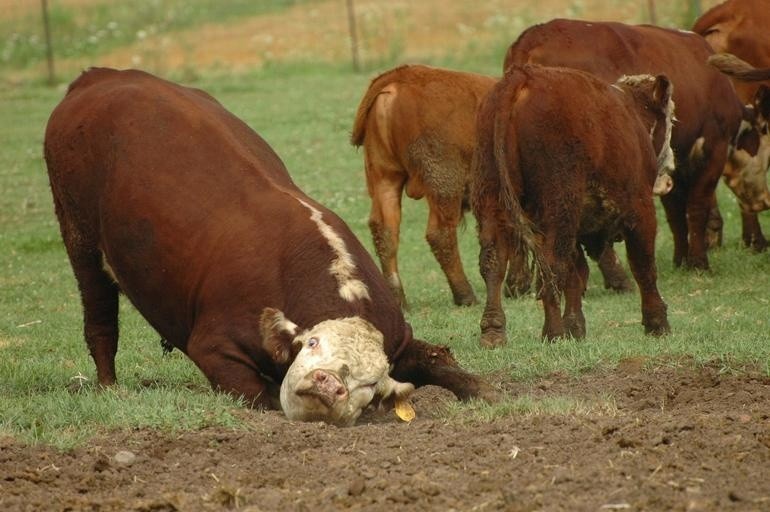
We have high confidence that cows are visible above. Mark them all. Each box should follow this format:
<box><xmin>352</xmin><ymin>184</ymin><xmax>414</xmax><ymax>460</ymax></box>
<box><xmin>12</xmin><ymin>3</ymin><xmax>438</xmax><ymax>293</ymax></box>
<box><xmin>350</xmin><ymin>65</ymin><xmax>503</xmax><ymax>309</ymax></box>
<box><xmin>502</xmin><ymin>0</ymin><xmax>767</xmax><ymax>274</ymax></box>
<box><xmin>470</xmin><ymin>67</ymin><xmax>678</xmax><ymax>350</ymax></box>
<box><xmin>40</xmin><ymin>64</ymin><xmax>508</xmax><ymax>430</ymax></box>
